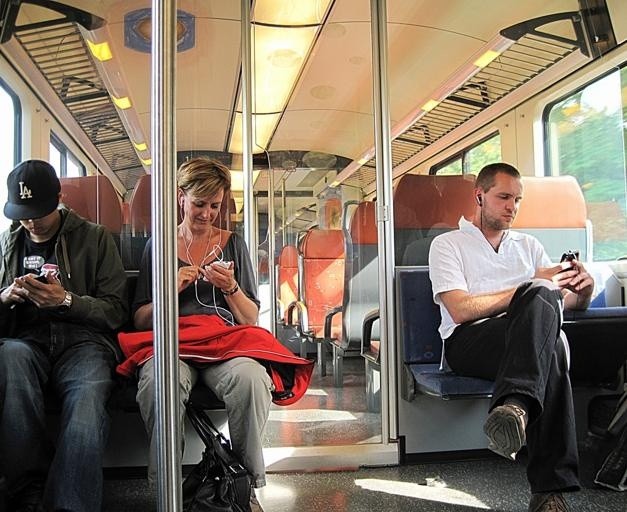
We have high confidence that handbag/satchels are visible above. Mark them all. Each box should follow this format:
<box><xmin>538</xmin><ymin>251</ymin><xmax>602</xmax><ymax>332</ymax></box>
<box><xmin>178</xmin><ymin>396</ymin><xmax>256</xmax><ymax>511</ymax></box>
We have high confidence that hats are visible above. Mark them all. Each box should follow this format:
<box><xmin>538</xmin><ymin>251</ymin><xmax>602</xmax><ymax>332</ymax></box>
<box><xmin>3</xmin><ymin>159</ymin><xmax>62</xmax><ymax>221</ymax></box>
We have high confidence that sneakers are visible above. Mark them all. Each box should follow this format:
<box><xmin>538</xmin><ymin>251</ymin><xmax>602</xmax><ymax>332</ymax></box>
<box><xmin>483</xmin><ymin>394</ymin><xmax>527</xmax><ymax>462</ymax></box>
<box><xmin>525</xmin><ymin>486</ymin><xmax>572</xmax><ymax>511</ymax></box>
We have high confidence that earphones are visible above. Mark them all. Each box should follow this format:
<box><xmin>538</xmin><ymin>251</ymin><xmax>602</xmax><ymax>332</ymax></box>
<box><xmin>181</xmin><ymin>197</ymin><xmax>184</xmax><ymax>208</ymax></box>
<box><xmin>476</xmin><ymin>196</ymin><xmax>482</xmax><ymax>206</ymax></box>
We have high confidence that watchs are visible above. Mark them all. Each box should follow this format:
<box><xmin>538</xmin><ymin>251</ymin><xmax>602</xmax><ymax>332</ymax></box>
<box><xmin>58</xmin><ymin>291</ymin><xmax>72</xmax><ymax>314</ymax></box>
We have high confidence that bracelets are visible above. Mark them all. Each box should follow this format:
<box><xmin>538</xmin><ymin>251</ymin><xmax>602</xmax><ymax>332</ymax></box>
<box><xmin>220</xmin><ymin>281</ymin><xmax>240</xmax><ymax>296</ymax></box>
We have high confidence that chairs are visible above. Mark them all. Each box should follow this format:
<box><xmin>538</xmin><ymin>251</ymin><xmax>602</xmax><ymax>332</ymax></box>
<box><xmin>255</xmin><ymin>174</ymin><xmax>595</xmax><ymax>406</ymax></box>
<box><xmin>51</xmin><ymin>175</ymin><xmax>238</xmax><ymax>405</ymax></box>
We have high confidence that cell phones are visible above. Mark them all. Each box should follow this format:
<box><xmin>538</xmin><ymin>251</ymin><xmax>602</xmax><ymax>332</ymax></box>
<box><xmin>24</xmin><ymin>275</ymin><xmax>49</xmax><ymax>290</ymax></box>
<box><xmin>558</xmin><ymin>251</ymin><xmax>579</xmax><ymax>280</ymax></box>
<box><xmin>203</xmin><ymin>261</ymin><xmax>231</xmax><ymax>281</ymax></box>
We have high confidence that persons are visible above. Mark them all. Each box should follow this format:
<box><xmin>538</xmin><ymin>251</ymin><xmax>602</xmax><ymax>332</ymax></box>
<box><xmin>428</xmin><ymin>162</ymin><xmax>595</xmax><ymax>512</ymax></box>
<box><xmin>132</xmin><ymin>155</ymin><xmax>276</xmax><ymax>512</ymax></box>
<box><xmin>0</xmin><ymin>158</ymin><xmax>129</xmax><ymax>511</ymax></box>
